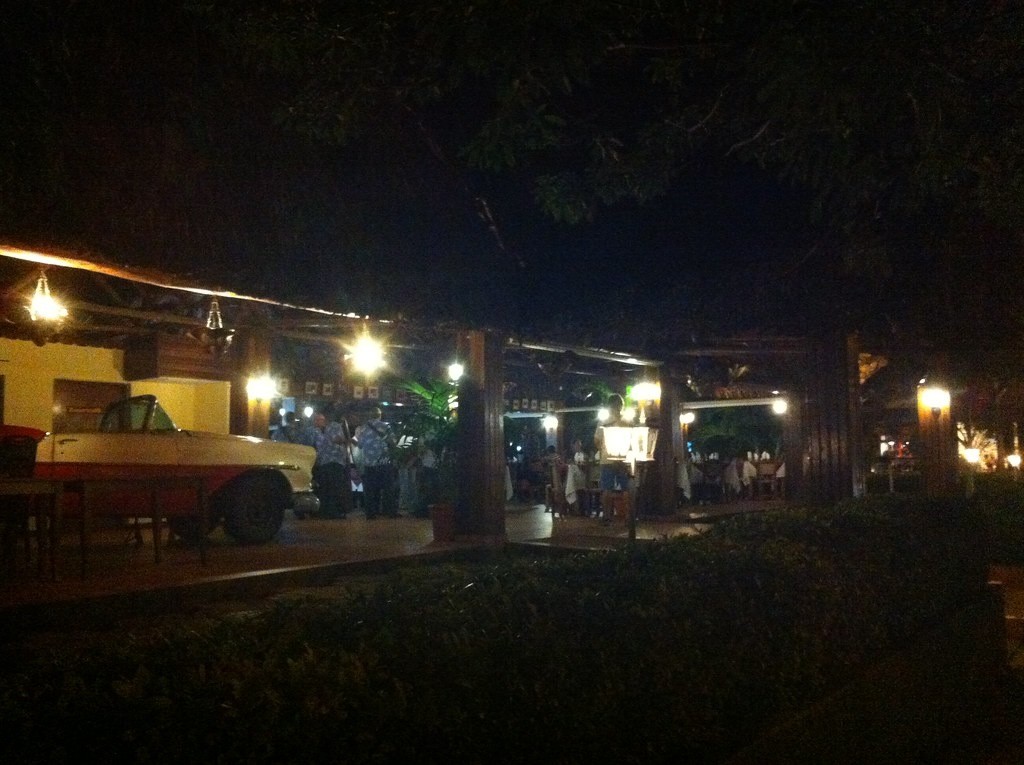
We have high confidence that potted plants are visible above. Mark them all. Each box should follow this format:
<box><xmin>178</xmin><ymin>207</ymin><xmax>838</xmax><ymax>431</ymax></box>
<box><xmin>385</xmin><ymin>446</ymin><xmax>426</xmax><ymax>516</ymax></box>
<box><xmin>394</xmin><ymin>379</ymin><xmax>467</xmax><ymax>505</ymax></box>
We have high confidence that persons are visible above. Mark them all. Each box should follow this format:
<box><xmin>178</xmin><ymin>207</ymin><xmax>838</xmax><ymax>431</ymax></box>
<box><xmin>544</xmin><ymin>444</ymin><xmax>562</xmax><ymax>480</ymax></box>
<box><xmin>272</xmin><ymin>412</ymin><xmax>307</xmax><ymax>445</ymax></box>
<box><xmin>347</xmin><ymin>426</ymin><xmax>365</xmax><ymax>480</ymax></box>
<box><xmin>519</xmin><ymin>423</ymin><xmax>543</xmax><ymax>505</ymax></box>
<box><xmin>594</xmin><ymin>394</ymin><xmax>638</xmax><ymax>524</ymax></box>
<box><xmin>297</xmin><ymin>412</ymin><xmax>326</xmax><ymax>450</ymax></box>
<box><xmin>312</xmin><ymin>408</ymin><xmax>348</xmax><ymax>519</ymax></box>
<box><xmin>689</xmin><ymin>452</ymin><xmax>758</xmax><ymax>505</ymax></box>
<box><xmin>356</xmin><ymin>407</ymin><xmax>402</xmax><ymax>520</ymax></box>
<box><xmin>564</xmin><ymin>439</ymin><xmax>583</xmax><ymax>465</ymax></box>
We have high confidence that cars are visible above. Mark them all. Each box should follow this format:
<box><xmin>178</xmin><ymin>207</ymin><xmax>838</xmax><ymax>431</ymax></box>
<box><xmin>0</xmin><ymin>394</ymin><xmax>325</xmax><ymax>550</ymax></box>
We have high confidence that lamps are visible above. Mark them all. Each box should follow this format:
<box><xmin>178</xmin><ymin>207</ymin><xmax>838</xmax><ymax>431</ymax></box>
<box><xmin>188</xmin><ymin>293</ymin><xmax>235</xmax><ymax>356</ymax></box>
<box><xmin>931</xmin><ymin>407</ymin><xmax>942</xmax><ymax>419</ymax></box>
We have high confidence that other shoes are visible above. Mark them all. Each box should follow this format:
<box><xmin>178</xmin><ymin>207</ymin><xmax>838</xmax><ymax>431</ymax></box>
<box><xmin>366</xmin><ymin>511</ymin><xmax>381</xmax><ymax>519</ymax></box>
<box><xmin>600</xmin><ymin>518</ymin><xmax>612</xmax><ymax>525</ymax></box>
<box><xmin>323</xmin><ymin>509</ymin><xmax>345</xmax><ymax>520</ymax></box>
<box><xmin>381</xmin><ymin>510</ymin><xmax>403</xmax><ymax>519</ymax></box>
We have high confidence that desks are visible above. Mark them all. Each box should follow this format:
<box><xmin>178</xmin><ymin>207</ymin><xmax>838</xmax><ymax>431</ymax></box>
<box><xmin>0</xmin><ymin>479</ymin><xmax>64</xmax><ymax>587</ymax></box>
<box><xmin>52</xmin><ymin>467</ymin><xmax>215</xmax><ymax>584</ymax></box>
